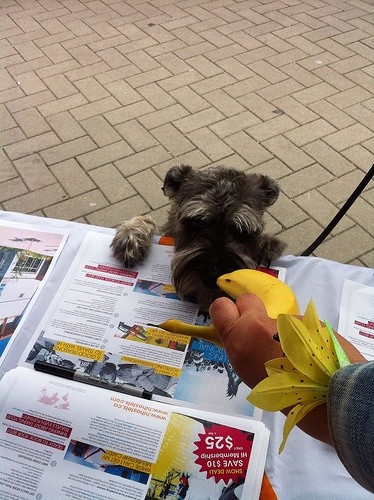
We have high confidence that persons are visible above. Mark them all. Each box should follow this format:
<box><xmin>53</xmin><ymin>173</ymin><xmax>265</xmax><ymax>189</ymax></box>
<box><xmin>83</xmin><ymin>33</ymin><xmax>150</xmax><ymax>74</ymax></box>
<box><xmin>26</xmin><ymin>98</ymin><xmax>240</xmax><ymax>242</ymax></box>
<box><xmin>148</xmin><ymin>346</ymin><xmax>246</xmax><ymax>500</ymax></box>
<box><xmin>208</xmin><ymin>292</ymin><xmax>374</xmax><ymax>493</ymax></box>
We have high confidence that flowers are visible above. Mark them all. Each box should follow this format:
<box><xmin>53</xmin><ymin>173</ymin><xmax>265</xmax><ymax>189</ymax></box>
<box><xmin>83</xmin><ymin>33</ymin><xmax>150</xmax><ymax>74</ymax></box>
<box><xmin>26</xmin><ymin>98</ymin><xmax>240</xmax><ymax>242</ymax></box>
<box><xmin>244</xmin><ymin>298</ymin><xmax>351</xmax><ymax>454</ymax></box>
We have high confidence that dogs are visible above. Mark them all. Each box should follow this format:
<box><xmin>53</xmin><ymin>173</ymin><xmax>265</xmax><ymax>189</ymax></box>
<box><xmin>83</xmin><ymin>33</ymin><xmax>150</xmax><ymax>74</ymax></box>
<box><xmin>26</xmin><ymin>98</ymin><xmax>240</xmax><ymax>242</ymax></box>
<box><xmin>108</xmin><ymin>162</ymin><xmax>289</xmax><ymax>314</ymax></box>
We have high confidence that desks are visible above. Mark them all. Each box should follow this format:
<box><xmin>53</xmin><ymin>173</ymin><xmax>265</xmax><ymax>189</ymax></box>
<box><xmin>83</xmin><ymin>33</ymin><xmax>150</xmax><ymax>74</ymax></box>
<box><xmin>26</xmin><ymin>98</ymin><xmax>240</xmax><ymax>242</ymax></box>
<box><xmin>1</xmin><ymin>210</ymin><xmax>374</xmax><ymax>500</ymax></box>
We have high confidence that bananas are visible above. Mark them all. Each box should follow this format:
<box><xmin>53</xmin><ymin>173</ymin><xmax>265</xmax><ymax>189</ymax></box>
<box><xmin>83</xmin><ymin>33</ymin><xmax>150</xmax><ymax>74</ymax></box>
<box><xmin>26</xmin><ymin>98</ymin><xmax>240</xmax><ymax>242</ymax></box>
<box><xmin>145</xmin><ymin>268</ymin><xmax>300</xmax><ymax>349</ymax></box>
